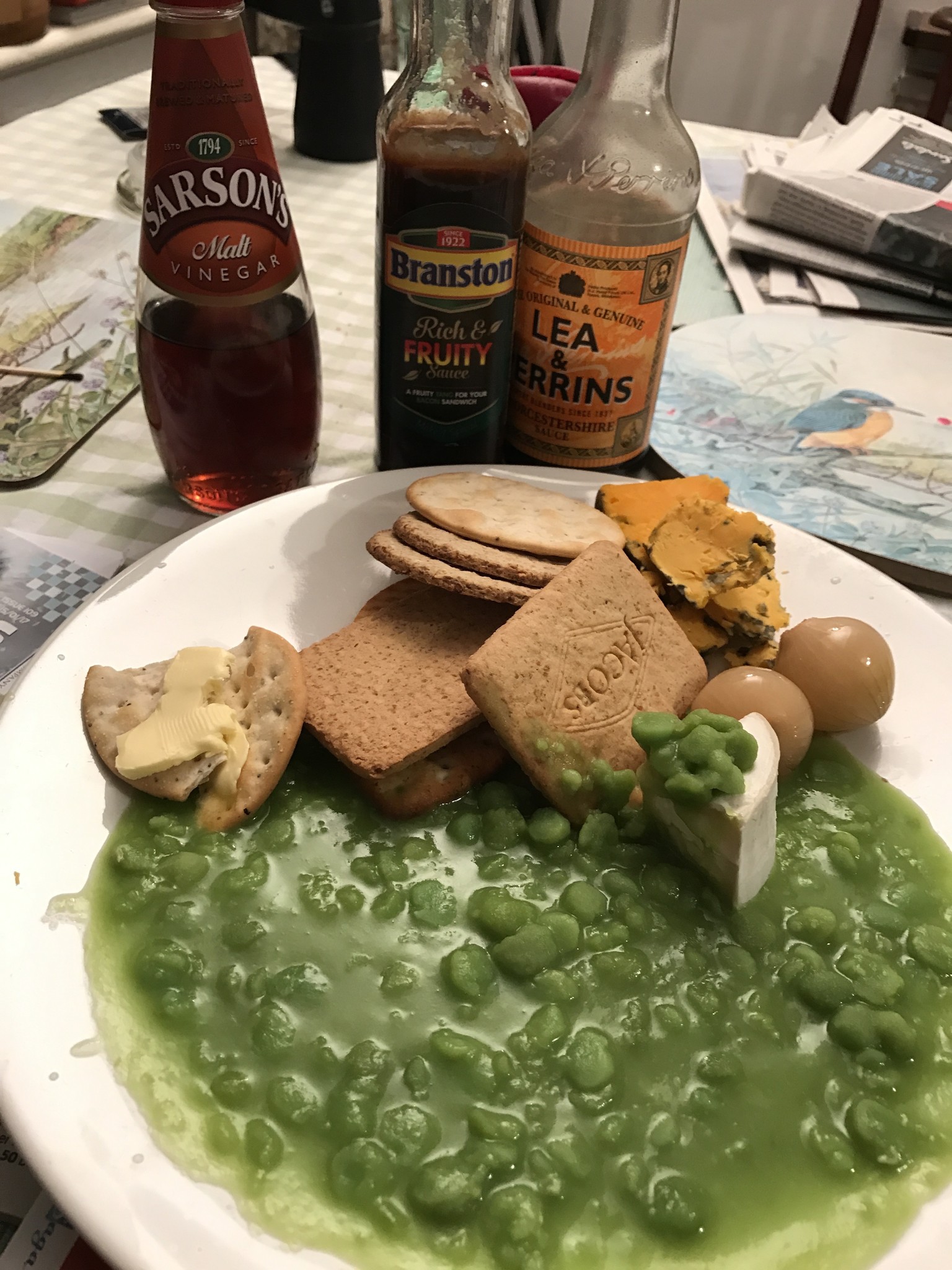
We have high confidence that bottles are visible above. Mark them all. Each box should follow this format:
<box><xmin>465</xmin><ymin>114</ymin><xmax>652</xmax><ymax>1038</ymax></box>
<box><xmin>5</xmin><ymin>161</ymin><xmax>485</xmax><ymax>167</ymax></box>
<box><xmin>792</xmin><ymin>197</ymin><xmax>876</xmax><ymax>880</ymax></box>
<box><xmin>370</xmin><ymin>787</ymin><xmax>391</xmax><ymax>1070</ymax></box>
<box><xmin>376</xmin><ymin>1</ymin><xmax>534</xmax><ymax>473</ymax></box>
<box><xmin>132</xmin><ymin>0</ymin><xmax>322</xmax><ymax>519</ymax></box>
<box><xmin>288</xmin><ymin>1</ymin><xmax>386</xmax><ymax>165</ymax></box>
<box><xmin>508</xmin><ymin>0</ymin><xmax>701</xmax><ymax>466</ymax></box>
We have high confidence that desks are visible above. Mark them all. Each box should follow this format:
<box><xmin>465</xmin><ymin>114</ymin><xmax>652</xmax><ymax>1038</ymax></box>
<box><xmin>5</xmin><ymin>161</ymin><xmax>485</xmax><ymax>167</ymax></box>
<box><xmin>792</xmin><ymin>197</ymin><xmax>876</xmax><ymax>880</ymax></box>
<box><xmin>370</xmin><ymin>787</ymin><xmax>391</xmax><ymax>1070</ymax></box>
<box><xmin>4</xmin><ymin>54</ymin><xmax>948</xmax><ymax>1269</ymax></box>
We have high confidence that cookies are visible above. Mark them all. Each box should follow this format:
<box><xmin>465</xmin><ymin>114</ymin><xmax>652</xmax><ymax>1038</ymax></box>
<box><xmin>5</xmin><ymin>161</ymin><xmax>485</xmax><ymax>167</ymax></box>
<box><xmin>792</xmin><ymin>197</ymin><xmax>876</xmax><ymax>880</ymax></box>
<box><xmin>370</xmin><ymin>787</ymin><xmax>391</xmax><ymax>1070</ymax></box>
<box><xmin>82</xmin><ymin>476</ymin><xmax>716</xmax><ymax>837</ymax></box>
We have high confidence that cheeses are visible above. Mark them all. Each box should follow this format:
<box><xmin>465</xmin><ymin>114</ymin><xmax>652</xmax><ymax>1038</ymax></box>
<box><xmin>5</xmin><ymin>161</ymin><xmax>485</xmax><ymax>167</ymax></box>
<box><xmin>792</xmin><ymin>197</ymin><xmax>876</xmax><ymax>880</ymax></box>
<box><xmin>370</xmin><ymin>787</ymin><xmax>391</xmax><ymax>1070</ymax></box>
<box><xmin>634</xmin><ymin>712</ymin><xmax>782</xmax><ymax>911</ymax></box>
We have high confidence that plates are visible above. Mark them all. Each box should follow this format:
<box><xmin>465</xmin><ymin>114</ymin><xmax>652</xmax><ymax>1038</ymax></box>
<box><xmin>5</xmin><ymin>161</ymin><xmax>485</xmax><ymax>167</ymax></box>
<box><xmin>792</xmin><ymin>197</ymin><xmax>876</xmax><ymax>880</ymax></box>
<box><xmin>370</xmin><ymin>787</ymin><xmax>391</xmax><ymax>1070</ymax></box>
<box><xmin>0</xmin><ymin>463</ymin><xmax>952</xmax><ymax>1270</ymax></box>
